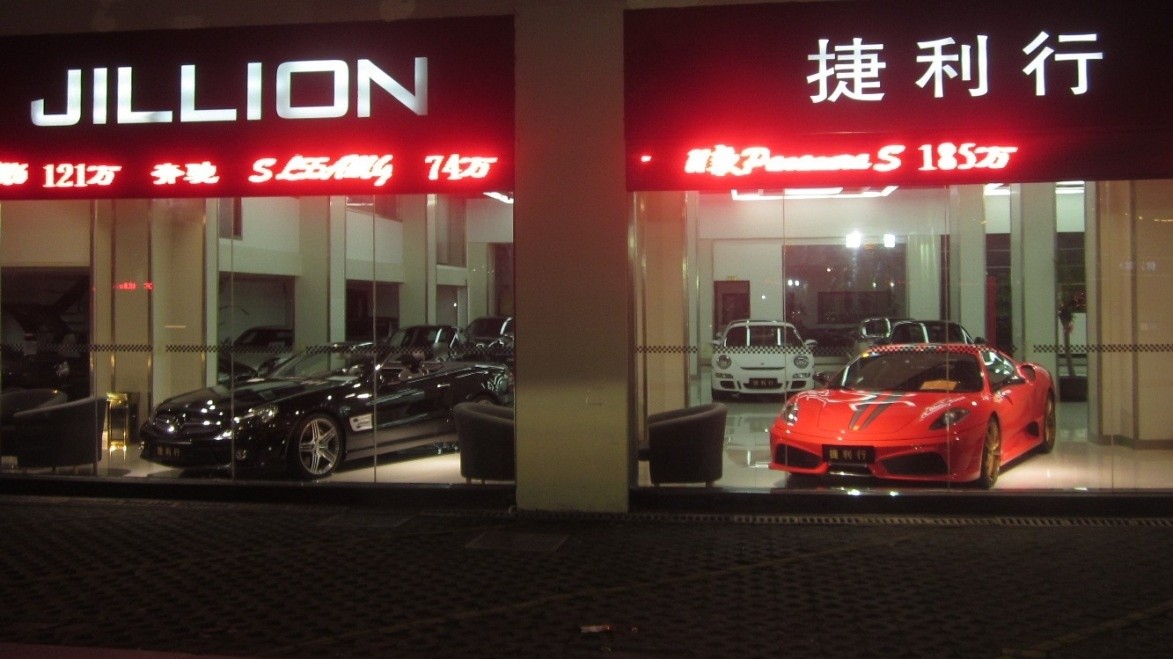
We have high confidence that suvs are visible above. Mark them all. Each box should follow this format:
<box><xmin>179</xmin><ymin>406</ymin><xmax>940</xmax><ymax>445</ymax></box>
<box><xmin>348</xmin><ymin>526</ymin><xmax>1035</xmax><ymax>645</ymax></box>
<box><xmin>460</xmin><ymin>313</ymin><xmax>516</xmax><ymax>359</ymax></box>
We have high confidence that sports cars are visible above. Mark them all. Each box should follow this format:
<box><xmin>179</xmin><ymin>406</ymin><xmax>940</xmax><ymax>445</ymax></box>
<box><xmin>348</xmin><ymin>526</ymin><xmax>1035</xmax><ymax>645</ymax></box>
<box><xmin>137</xmin><ymin>343</ymin><xmax>513</xmax><ymax>482</ymax></box>
<box><xmin>768</xmin><ymin>340</ymin><xmax>1056</xmax><ymax>492</ymax></box>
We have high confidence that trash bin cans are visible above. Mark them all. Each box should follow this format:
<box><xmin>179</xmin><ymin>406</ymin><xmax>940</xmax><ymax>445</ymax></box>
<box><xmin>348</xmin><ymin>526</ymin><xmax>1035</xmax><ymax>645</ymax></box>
<box><xmin>105</xmin><ymin>390</ymin><xmax>140</xmax><ymax>449</ymax></box>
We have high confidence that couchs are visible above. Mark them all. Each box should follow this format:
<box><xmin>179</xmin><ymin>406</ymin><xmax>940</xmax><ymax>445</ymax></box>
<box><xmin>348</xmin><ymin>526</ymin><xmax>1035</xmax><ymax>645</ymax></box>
<box><xmin>12</xmin><ymin>394</ymin><xmax>107</xmax><ymax>475</ymax></box>
<box><xmin>645</xmin><ymin>401</ymin><xmax>727</xmax><ymax>491</ymax></box>
<box><xmin>1</xmin><ymin>388</ymin><xmax>68</xmax><ymax>468</ymax></box>
<box><xmin>452</xmin><ymin>401</ymin><xmax>514</xmax><ymax>492</ymax></box>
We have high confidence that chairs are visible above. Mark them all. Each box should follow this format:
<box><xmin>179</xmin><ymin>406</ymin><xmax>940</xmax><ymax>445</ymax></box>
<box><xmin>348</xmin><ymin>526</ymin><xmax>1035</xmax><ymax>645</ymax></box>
<box><xmin>348</xmin><ymin>348</ymin><xmax>429</xmax><ymax>389</ymax></box>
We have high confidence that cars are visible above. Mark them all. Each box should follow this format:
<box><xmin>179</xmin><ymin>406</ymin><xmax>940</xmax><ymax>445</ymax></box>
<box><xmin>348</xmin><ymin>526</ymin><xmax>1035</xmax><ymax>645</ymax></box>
<box><xmin>216</xmin><ymin>327</ymin><xmax>298</xmax><ymax>382</ymax></box>
<box><xmin>841</xmin><ymin>317</ymin><xmax>986</xmax><ymax>366</ymax></box>
<box><xmin>706</xmin><ymin>316</ymin><xmax>816</xmax><ymax>400</ymax></box>
<box><xmin>374</xmin><ymin>325</ymin><xmax>472</xmax><ymax>362</ymax></box>
